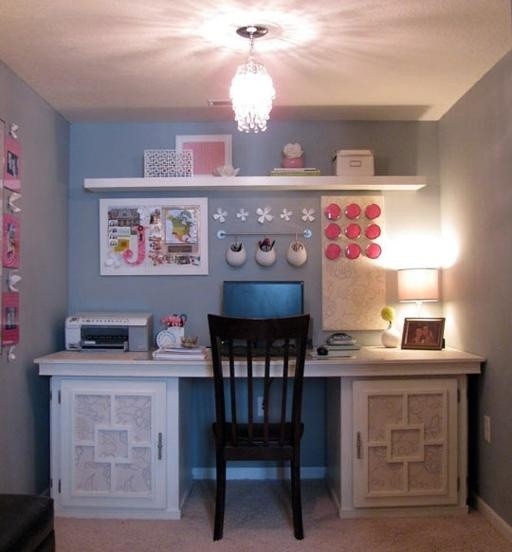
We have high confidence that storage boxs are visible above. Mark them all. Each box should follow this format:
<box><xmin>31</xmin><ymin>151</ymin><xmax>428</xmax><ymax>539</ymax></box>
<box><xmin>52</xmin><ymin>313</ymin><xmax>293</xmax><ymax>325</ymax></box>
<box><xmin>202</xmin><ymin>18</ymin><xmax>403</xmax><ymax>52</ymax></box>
<box><xmin>333</xmin><ymin>150</ymin><xmax>374</xmax><ymax>176</ymax></box>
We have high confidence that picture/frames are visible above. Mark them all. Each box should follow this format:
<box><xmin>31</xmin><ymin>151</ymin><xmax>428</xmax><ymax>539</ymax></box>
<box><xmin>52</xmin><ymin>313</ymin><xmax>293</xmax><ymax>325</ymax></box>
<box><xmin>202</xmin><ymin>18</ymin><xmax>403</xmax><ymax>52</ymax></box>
<box><xmin>401</xmin><ymin>318</ymin><xmax>445</xmax><ymax>350</ymax></box>
<box><xmin>175</xmin><ymin>134</ymin><xmax>232</xmax><ymax>178</ymax></box>
<box><xmin>1</xmin><ymin>134</ymin><xmax>21</xmax><ymax>347</ymax></box>
<box><xmin>98</xmin><ymin>197</ymin><xmax>209</xmax><ymax>276</ymax></box>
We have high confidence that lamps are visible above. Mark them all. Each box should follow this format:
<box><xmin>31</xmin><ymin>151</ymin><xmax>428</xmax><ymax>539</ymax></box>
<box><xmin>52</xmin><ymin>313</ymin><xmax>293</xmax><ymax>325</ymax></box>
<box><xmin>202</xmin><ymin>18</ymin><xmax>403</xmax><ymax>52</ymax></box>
<box><xmin>397</xmin><ymin>269</ymin><xmax>439</xmax><ymax>317</ymax></box>
<box><xmin>230</xmin><ymin>26</ymin><xmax>275</xmax><ymax>132</ymax></box>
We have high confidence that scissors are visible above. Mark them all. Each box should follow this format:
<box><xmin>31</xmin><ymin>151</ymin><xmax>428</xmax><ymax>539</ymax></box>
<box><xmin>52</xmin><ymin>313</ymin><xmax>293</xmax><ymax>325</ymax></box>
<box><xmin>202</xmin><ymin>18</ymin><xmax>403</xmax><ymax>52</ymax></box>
<box><xmin>173</xmin><ymin>314</ymin><xmax>187</xmax><ymax>326</ymax></box>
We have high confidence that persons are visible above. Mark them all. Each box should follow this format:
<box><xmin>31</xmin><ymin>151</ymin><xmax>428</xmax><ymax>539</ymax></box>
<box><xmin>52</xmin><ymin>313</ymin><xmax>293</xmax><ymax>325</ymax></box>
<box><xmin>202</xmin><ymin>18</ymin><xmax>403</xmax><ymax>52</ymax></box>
<box><xmin>411</xmin><ymin>326</ymin><xmax>436</xmax><ymax>344</ymax></box>
<box><xmin>7</xmin><ymin>313</ymin><xmax>12</xmax><ymax>328</ymax></box>
<box><xmin>6</xmin><ymin>150</ymin><xmax>13</xmax><ymax>174</ymax></box>
<box><xmin>11</xmin><ymin>158</ymin><xmax>16</xmax><ymax>177</ymax></box>
<box><xmin>11</xmin><ymin>311</ymin><xmax>16</xmax><ymax>328</ymax></box>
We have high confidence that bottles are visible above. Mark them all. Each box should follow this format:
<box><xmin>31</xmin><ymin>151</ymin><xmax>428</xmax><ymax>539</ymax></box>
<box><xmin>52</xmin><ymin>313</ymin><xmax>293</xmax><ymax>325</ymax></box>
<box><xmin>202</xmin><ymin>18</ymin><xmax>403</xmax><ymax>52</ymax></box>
<box><xmin>285</xmin><ymin>241</ymin><xmax>308</xmax><ymax>266</ymax></box>
<box><xmin>255</xmin><ymin>247</ymin><xmax>276</xmax><ymax>267</ymax></box>
<box><xmin>225</xmin><ymin>243</ymin><xmax>247</xmax><ymax>268</ymax></box>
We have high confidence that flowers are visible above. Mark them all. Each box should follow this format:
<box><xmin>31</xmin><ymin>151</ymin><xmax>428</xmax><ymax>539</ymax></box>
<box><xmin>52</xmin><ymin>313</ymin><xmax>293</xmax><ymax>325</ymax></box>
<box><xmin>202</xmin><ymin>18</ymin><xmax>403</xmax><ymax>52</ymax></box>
<box><xmin>160</xmin><ymin>315</ymin><xmax>183</xmax><ymax>328</ymax></box>
<box><xmin>282</xmin><ymin>142</ymin><xmax>303</xmax><ymax>159</ymax></box>
<box><xmin>381</xmin><ymin>306</ymin><xmax>395</xmax><ymax>324</ymax></box>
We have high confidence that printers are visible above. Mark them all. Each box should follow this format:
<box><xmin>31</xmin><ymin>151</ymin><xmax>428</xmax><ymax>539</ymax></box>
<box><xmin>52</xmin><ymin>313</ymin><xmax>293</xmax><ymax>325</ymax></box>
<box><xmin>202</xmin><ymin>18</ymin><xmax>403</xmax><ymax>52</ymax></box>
<box><xmin>63</xmin><ymin>311</ymin><xmax>154</xmax><ymax>354</ymax></box>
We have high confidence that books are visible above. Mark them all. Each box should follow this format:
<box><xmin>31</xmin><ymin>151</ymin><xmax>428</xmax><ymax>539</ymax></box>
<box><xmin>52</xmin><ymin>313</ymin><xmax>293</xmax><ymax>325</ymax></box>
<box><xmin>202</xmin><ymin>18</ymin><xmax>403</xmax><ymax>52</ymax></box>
<box><xmin>307</xmin><ymin>336</ymin><xmax>360</xmax><ymax>360</ymax></box>
<box><xmin>270</xmin><ymin>166</ymin><xmax>323</xmax><ymax>176</ymax></box>
<box><xmin>152</xmin><ymin>345</ymin><xmax>209</xmax><ymax>361</ymax></box>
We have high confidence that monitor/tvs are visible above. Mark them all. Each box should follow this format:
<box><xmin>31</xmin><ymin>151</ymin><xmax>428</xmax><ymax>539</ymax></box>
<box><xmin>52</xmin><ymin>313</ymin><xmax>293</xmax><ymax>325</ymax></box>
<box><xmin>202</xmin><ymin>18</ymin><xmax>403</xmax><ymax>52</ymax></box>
<box><xmin>221</xmin><ymin>280</ymin><xmax>304</xmax><ymax>317</ymax></box>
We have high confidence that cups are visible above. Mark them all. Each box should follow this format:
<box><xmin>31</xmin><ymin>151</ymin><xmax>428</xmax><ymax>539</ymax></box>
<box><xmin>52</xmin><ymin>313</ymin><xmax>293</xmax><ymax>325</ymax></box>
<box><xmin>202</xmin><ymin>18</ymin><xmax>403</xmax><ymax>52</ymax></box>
<box><xmin>181</xmin><ymin>336</ymin><xmax>199</xmax><ymax>348</ymax></box>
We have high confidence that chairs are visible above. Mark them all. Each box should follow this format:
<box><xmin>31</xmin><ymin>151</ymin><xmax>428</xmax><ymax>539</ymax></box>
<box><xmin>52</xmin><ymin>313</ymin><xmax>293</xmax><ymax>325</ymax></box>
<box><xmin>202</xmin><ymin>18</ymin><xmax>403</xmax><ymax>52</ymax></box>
<box><xmin>207</xmin><ymin>312</ymin><xmax>311</xmax><ymax>540</ymax></box>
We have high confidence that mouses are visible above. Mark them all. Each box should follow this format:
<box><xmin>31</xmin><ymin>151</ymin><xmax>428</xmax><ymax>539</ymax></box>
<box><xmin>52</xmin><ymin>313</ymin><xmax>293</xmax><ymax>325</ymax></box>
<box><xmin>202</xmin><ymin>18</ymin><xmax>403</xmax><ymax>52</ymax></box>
<box><xmin>317</xmin><ymin>347</ymin><xmax>330</xmax><ymax>355</ymax></box>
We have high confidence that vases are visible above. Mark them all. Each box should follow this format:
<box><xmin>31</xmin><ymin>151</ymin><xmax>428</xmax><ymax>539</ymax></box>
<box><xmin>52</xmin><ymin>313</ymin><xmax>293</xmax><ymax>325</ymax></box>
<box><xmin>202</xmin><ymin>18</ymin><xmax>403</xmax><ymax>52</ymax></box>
<box><xmin>282</xmin><ymin>157</ymin><xmax>304</xmax><ymax>168</ymax></box>
<box><xmin>382</xmin><ymin>324</ymin><xmax>401</xmax><ymax>348</ymax></box>
<box><xmin>168</xmin><ymin>327</ymin><xmax>184</xmax><ymax>347</ymax></box>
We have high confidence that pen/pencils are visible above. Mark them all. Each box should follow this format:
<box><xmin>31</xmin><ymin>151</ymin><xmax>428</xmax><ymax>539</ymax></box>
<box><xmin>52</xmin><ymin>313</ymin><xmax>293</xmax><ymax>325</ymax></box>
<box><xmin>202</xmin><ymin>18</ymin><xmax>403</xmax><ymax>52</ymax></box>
<box><xmin>231</xmin><ymin>242</ymin><xmax>242</xmax><ymax>252</ymax></box>
<box><xmin>293</xmin><ymin>232</ymin><xmax>302</xmax><ymax>251</ymax></box>
<box><xmin>258</xmin><ymin>238</ymin><xmax>275</xmax><ymax>252</ymax></box>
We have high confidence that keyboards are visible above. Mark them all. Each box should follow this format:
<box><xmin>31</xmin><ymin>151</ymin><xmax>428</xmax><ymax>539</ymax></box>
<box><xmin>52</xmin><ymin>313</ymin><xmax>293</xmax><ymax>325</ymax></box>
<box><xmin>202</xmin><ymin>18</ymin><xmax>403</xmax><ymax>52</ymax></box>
<box><xmin>217</xmin><ymin>344</ymin><xmax>304</xmax><ymax>358</ymax></box>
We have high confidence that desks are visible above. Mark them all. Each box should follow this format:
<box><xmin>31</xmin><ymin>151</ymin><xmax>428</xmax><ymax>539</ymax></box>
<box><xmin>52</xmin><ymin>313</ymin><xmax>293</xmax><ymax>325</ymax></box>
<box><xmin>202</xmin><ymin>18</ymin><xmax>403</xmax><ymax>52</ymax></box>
<box><xmin>34</xmin><ymin>344</ymin><xmax>488</xmax><ymax>520</ymax></box>
